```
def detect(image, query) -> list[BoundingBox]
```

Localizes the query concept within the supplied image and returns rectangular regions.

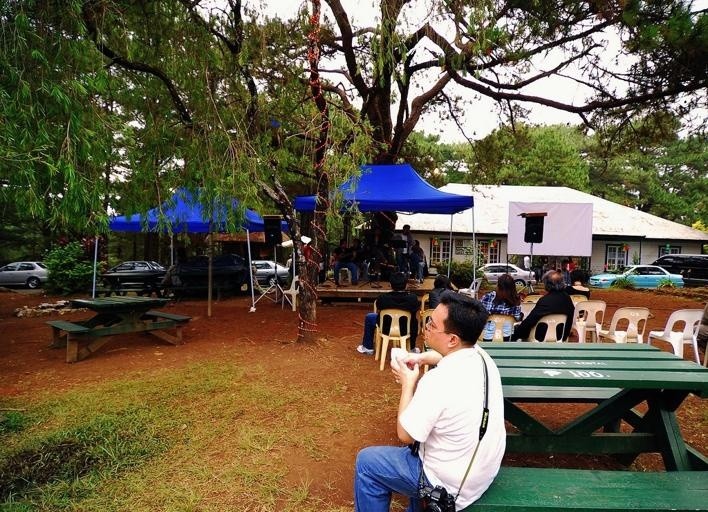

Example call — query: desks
[97,271,166,297]
[59,296,177,361]
[423,341,708,471]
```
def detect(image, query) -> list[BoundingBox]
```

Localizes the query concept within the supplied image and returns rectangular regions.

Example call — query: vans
[649,253,708,286]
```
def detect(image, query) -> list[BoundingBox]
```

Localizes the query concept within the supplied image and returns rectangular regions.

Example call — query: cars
[476,263,537,286]
[101,261,167,283]
[0,261,51,288]
[250,260,291,287]
[589,266,685,293]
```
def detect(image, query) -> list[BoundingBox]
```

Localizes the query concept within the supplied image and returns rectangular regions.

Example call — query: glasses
[428,317,454,334]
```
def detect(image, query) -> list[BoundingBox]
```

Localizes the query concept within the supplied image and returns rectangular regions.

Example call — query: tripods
[517,243,537,293]
[252,244,293,307]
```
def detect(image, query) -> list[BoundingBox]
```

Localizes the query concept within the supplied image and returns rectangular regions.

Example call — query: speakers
[524,216,544,243]
[263,216,283,244]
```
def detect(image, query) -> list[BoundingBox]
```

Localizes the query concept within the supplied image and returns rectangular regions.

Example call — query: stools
[339,267,352,285]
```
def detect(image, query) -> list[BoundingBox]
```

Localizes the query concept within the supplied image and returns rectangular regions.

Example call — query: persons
[285,225,591,356]
[693,302,708,355]
[355,288,507,512]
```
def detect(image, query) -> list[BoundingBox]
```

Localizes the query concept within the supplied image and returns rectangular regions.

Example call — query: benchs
[121,282,170,288]
[459,467,708,512]
[501,384,622,404]
[87,288,152,297]
[44,320,90,363]
[146,311,192,345]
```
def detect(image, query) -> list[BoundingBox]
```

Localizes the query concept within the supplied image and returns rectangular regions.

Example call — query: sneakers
[357,345,374,355]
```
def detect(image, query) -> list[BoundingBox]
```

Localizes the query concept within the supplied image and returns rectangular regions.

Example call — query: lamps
[489,240,494,247]
[434,237,438,245]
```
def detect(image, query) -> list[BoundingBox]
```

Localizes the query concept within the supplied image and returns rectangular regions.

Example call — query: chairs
[571,300,607,342]
[527,314,567,342]
[420,309,434,332]
[414,279,588,310]
[281,275,300,311]
[479,314,516,343]
[519,302,535,320]
[600,306,650,344]
[373,309,411,372]
[651,309,704,365]
